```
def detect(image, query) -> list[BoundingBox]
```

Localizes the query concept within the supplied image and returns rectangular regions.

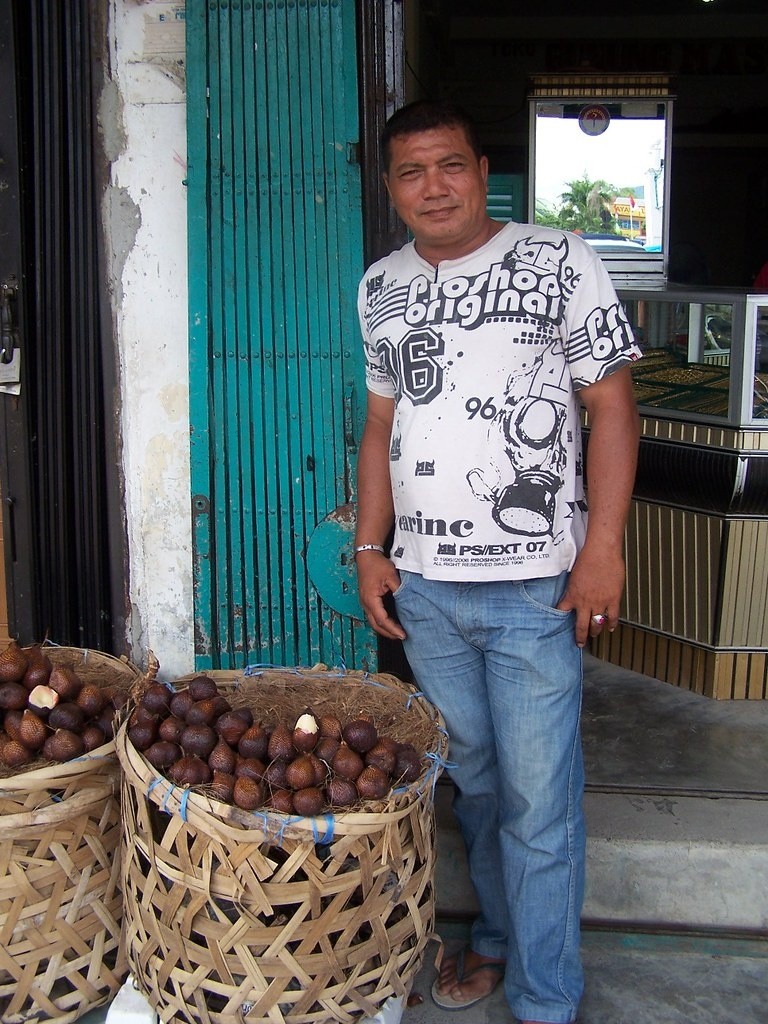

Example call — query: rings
[592,615,604,625]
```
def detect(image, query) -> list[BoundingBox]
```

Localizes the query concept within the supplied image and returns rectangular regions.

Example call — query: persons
[355,98,642,1024]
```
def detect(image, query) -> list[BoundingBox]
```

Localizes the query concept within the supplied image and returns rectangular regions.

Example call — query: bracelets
[354,544,385,555]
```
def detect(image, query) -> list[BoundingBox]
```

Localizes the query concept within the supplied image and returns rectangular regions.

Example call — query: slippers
[430,943,507,1010]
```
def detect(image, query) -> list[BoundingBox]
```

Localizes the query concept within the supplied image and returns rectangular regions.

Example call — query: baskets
[0,646,143,1024]
[120,667,448,1024]
